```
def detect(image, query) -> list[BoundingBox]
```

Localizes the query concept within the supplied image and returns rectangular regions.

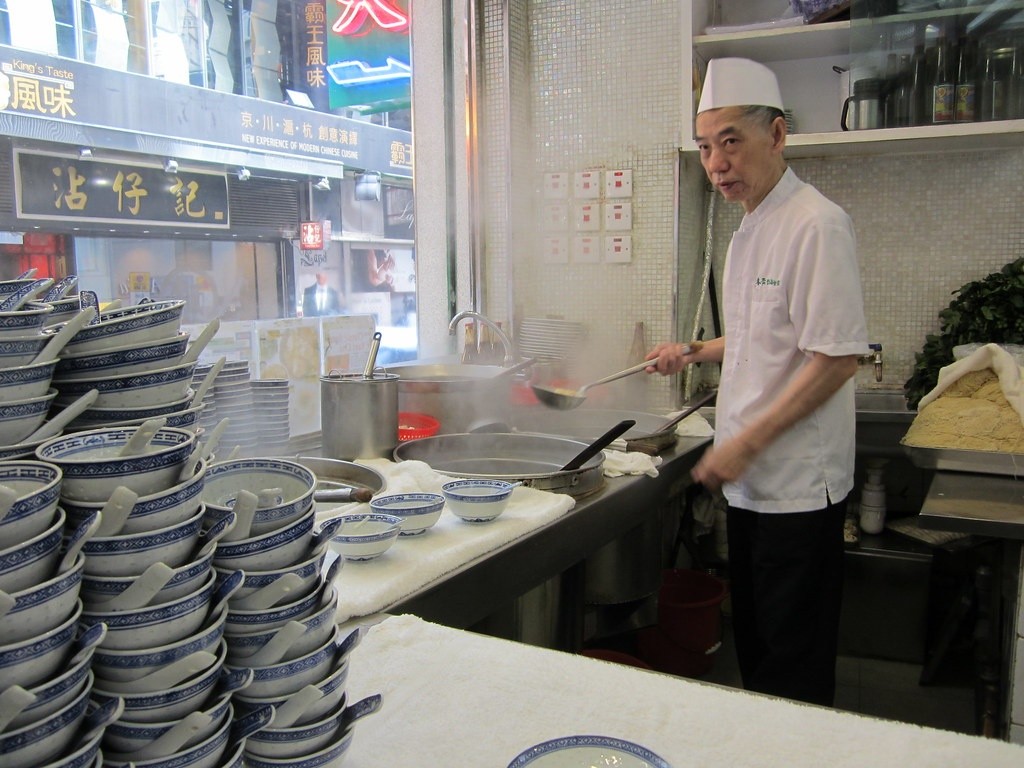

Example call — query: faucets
[446,309,517,368]
[870,343,886,382]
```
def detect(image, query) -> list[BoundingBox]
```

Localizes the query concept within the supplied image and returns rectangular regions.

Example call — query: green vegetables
[902,256,1024,412]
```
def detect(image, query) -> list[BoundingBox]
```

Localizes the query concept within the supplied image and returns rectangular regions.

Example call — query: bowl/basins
[0,277,669,768]
[839,65,878,130]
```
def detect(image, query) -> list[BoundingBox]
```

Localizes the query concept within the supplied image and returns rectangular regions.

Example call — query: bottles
[887,36,1024,126]
[493,321,503,366]
[625,321,648,408]
[460,321,479,366]
[478,322,494,365]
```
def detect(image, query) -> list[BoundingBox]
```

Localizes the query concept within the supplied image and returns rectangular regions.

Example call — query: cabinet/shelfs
[681,0,1024,163]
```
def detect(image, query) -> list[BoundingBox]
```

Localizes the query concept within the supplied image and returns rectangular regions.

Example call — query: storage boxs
[836,511,1001,668]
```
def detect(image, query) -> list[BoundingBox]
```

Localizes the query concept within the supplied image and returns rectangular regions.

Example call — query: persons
[642,57,871,710]
[352,249,399,292]
[302,270,340,316]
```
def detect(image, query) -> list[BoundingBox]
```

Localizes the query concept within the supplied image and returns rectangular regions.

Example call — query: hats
[697,57,785,115]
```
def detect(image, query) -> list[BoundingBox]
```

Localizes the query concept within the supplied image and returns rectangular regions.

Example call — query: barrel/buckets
[580,649,654,671]
[637,568,723,676]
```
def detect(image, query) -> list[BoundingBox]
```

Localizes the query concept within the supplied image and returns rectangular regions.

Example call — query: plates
[519,316,585,389]
[783,107,794,133]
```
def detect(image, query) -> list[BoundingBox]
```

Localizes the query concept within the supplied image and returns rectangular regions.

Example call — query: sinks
[684,389,926,514]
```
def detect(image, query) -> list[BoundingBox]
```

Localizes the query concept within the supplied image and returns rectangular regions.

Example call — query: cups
[840,77,886,131]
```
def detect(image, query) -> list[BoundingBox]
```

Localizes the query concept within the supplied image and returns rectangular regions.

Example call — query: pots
[374,363,513,423]
[319,365,400,462]
[392,431,606,501]
[511,408,678,455]
